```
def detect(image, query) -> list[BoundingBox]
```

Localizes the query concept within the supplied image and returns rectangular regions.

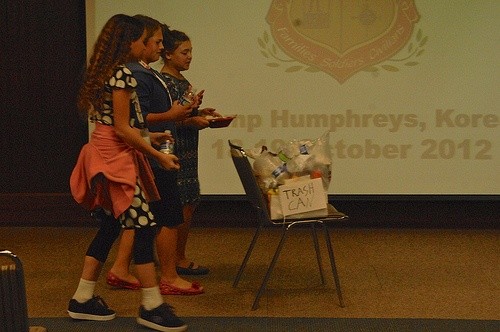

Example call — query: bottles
[256,153,289,185]
[159,130,173,169]
[178,88,196,106]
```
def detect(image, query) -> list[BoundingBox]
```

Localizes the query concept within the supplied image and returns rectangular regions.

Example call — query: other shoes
[160,278,204,295]
[104,270,140,290]
[176,261,210,274]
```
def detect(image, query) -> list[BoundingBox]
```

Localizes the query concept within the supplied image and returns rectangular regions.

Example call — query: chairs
[229,139,350,312]
[0,251,47,332]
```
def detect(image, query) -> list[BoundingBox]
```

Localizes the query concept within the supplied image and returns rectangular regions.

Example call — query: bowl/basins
[206,117,236,128]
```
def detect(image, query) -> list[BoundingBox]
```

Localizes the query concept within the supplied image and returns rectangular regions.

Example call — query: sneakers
[136,303,187,331]
[67,296,116,321]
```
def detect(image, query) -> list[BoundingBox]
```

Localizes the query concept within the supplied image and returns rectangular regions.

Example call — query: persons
[105,15,205,296]
[66,14,188,332]
[133,22,222,276]
